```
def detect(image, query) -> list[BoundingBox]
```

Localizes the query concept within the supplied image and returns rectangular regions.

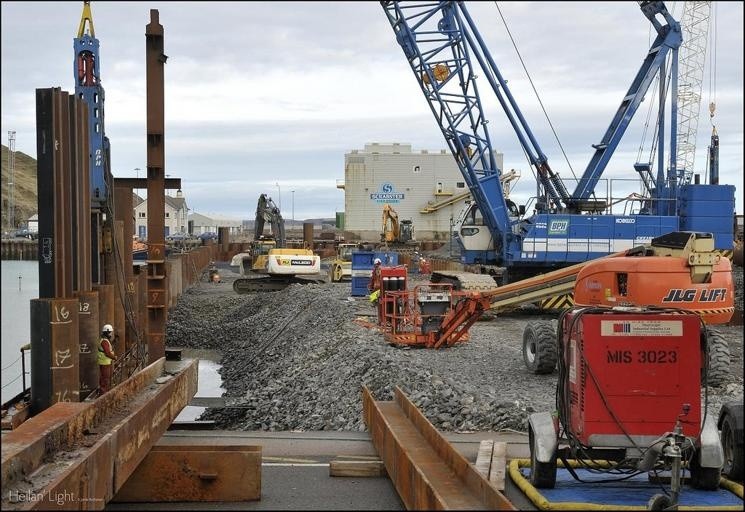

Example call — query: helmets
[374,258,382,265]
[102,324,114,332]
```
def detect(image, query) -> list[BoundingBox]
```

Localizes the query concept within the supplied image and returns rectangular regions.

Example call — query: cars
[166,231,190,242]
[195,232,217,240]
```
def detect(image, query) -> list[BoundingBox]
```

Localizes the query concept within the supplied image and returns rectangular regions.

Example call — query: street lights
[275,184,282,211]
[134,168,140,205]
[290,189,294,234]
[166,174,170,195]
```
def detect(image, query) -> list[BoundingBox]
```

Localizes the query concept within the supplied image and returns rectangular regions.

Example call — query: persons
[370,257,382,294]
[99,323,118,397]
[410,251,419,275]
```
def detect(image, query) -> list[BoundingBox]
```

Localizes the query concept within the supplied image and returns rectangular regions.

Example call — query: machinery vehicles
[329,243,367,283]
[376,0,745,511]
[232,193,327,295]
